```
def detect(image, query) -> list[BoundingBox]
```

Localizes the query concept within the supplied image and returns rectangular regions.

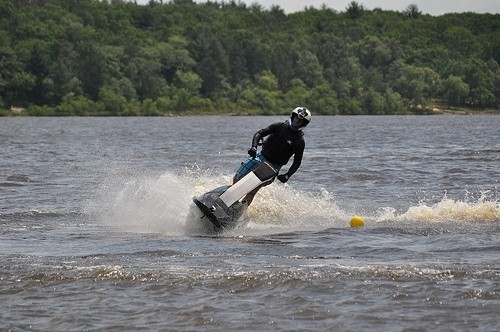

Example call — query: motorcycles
[193,149,286,231]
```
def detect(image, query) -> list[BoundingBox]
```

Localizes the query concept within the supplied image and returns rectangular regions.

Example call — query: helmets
[289,106,311,130]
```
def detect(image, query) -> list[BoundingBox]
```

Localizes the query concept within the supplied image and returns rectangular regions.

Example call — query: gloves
[248,146,257,160]
[277,174,288,183]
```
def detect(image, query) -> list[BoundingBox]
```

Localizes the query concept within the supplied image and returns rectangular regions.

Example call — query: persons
[233,107,311,209]
